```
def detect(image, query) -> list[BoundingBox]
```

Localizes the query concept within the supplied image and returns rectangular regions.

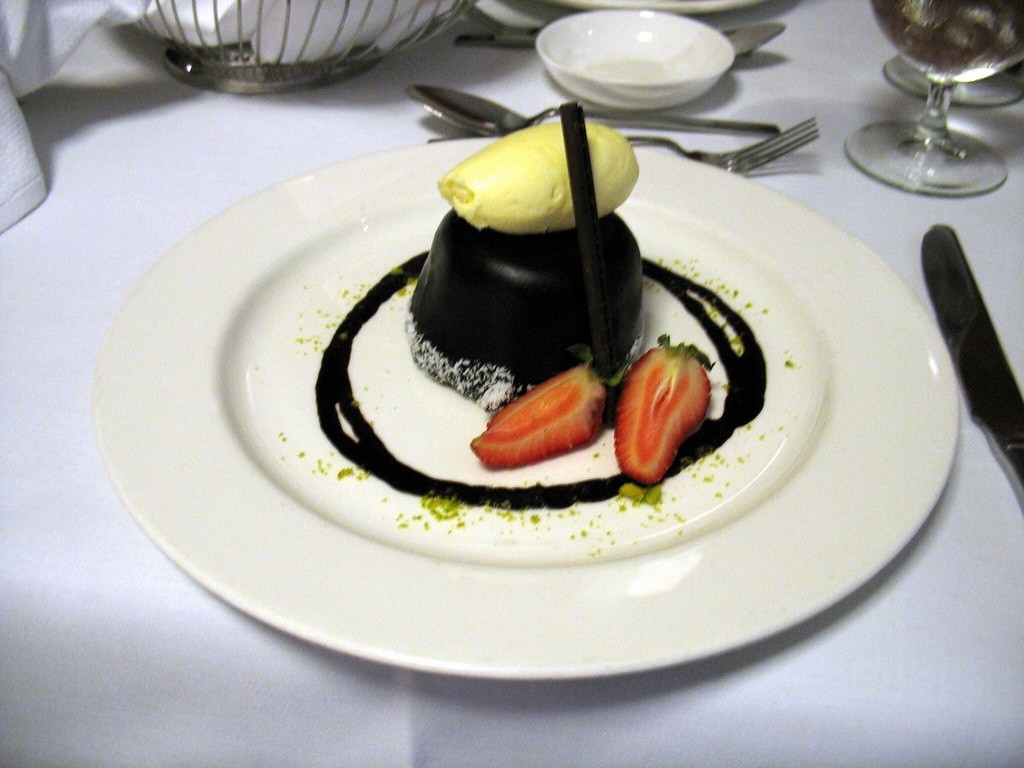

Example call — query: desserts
[405,101,645,414]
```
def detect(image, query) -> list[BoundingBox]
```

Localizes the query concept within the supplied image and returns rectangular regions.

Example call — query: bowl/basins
[535,9,735,107]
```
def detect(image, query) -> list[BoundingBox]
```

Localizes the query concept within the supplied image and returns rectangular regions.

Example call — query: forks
[627,116,819,171]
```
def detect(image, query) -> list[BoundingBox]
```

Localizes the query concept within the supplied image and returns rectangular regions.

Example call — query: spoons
[455,22,785,58]
[408,85,779,142]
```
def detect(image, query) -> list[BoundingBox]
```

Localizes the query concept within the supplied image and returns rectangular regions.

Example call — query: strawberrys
[469,344,635,469]
[615,333,716,484]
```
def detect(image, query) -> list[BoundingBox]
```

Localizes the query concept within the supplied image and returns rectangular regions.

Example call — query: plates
[92,140,959,683]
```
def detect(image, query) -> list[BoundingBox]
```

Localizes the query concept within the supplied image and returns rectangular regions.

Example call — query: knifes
[920,223,1024,500]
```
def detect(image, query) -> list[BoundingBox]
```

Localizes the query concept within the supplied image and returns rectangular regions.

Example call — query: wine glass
[844,0,1024,196]
[881,54,1024,109]
